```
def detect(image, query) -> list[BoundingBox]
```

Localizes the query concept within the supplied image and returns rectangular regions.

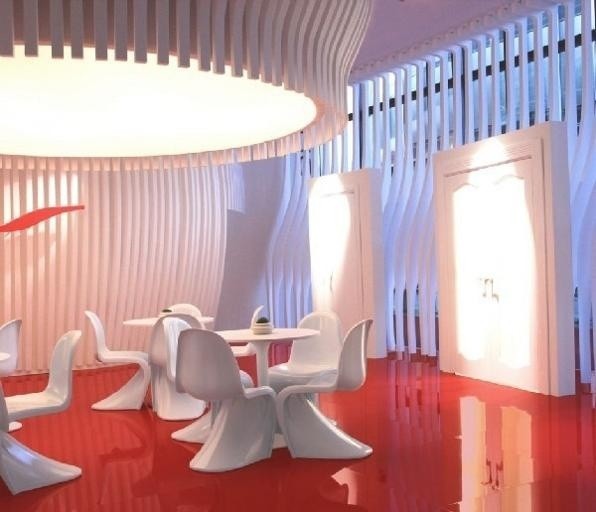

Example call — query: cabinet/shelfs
[317,361,391,508]
[450,391,550,509]
[433,121,575,397]
[308,167,387,359]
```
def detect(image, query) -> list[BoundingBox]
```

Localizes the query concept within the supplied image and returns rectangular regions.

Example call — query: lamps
[2,0,374,172]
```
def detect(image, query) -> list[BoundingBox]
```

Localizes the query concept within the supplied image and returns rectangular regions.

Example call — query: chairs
[148,313,208,421]
[84,309,151,410]
[1,318,23,431]
[229,305,268,358]
[1,329,83,495]
[274,318,374,461]
[170,328,277,473]
[266,309,346,426]
[160,303,201,317]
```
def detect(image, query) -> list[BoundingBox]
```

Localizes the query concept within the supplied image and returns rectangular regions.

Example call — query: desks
[213,326,321,451]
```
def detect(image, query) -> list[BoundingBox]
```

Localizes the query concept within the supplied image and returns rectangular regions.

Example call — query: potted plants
[252,317,272,334]
